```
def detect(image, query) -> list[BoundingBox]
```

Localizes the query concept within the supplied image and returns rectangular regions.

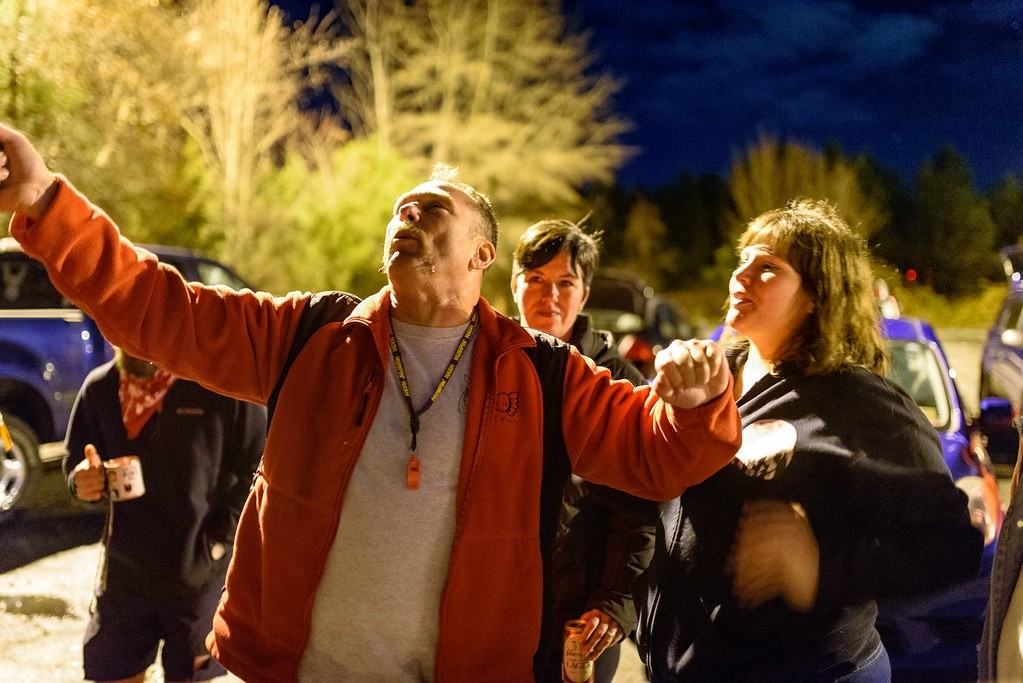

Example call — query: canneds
[561,620,596,683]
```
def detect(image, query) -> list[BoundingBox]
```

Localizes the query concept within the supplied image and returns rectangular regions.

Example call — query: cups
[98,456,145,502]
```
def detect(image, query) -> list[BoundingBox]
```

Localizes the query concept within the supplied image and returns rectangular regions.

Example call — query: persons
[632,194,985,683]
[0,122,744,683]
[503,214,657,683]
[974,393,1023,683]
[63,337,267,683]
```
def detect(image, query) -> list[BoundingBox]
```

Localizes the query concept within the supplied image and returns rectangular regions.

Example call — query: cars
[579,274,701,379]
[876,317,1020,682]
[979,283,1023,423]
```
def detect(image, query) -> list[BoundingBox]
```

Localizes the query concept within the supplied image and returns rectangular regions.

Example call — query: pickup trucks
[0,236,260,525]
